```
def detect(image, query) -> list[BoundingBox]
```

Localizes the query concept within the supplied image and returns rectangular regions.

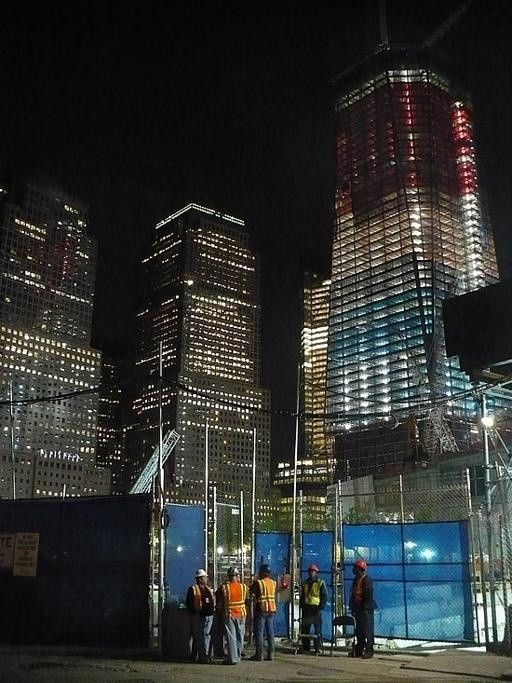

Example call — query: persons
[186,567,216,664]
[215,567,251,665]
[347,561,378,659]
[296,564,328,654]
[249,564,278,661]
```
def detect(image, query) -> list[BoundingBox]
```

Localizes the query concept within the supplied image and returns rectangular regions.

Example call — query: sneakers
[249,653,274,661]
[293,646,322,654]
[197,657,236,665]
[348,649,373,660]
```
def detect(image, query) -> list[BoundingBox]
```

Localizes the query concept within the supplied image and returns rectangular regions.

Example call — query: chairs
[293,615,356,657]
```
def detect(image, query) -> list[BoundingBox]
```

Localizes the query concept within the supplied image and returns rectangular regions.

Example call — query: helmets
[355,560,367,570]
[260,565,271,572]
[195,568,208,577]
[308,564,318,571]
[227,567,240,575]
